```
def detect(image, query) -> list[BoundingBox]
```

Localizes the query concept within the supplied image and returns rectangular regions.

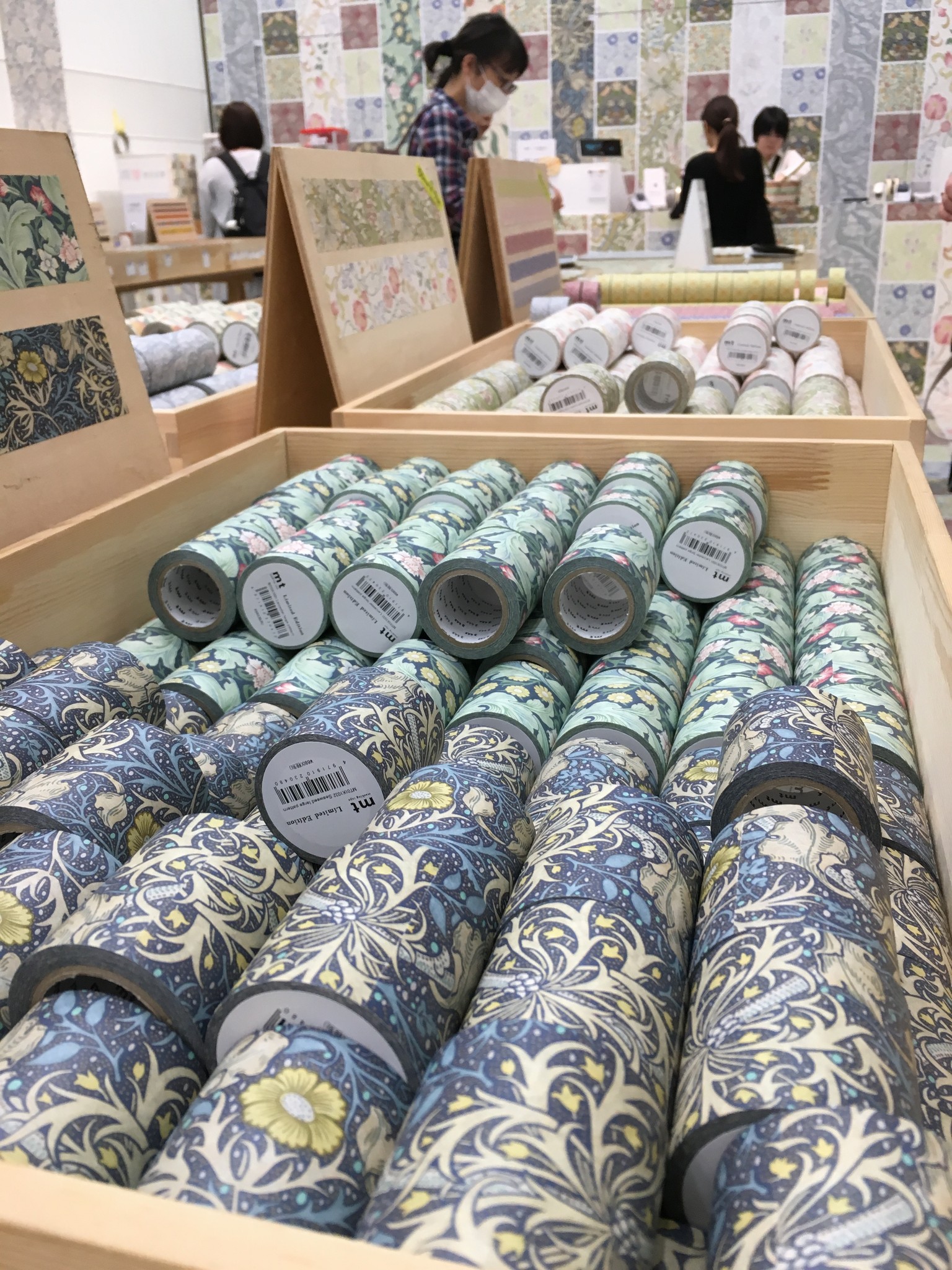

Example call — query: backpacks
[214,150,270,237]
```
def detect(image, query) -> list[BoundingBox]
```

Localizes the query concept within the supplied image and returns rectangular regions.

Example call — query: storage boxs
[0,128,952,1270]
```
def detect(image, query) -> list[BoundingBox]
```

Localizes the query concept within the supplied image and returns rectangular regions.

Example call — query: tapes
[124,300,266,408]
[0,451,949,1270]
[416,265,860,417]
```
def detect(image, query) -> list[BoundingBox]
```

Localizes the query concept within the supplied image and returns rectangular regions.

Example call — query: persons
[667,95,791,246]
[194,102,273,305]
[409,11,530,270]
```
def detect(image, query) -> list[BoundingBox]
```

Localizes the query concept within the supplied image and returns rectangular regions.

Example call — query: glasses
[483,57,518,96]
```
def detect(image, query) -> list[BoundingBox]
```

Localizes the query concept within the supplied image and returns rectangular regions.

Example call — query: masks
[463,78,509,116]
